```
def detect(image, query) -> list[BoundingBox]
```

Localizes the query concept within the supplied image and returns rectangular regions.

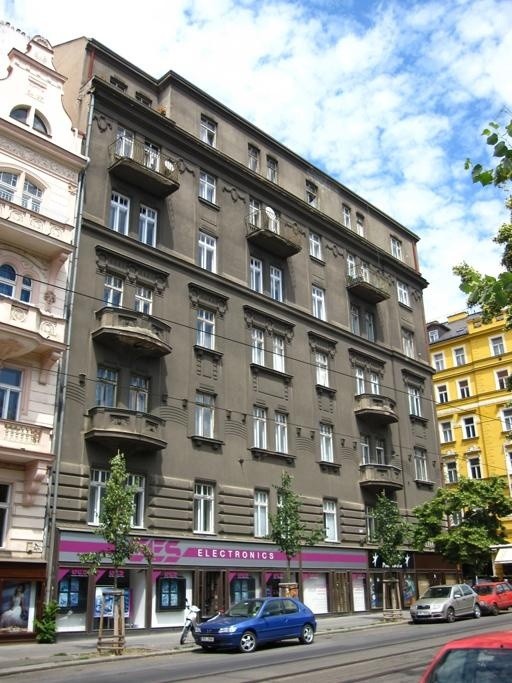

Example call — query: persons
[0,584,27,628]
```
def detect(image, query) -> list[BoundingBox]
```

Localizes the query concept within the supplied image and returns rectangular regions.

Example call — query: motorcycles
[177,596,223,645]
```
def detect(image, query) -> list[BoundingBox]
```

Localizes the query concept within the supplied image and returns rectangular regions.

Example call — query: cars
[419,631,512,682]
[410,582,481,623]
[470,580,512,616]
[195,598,317,652]
